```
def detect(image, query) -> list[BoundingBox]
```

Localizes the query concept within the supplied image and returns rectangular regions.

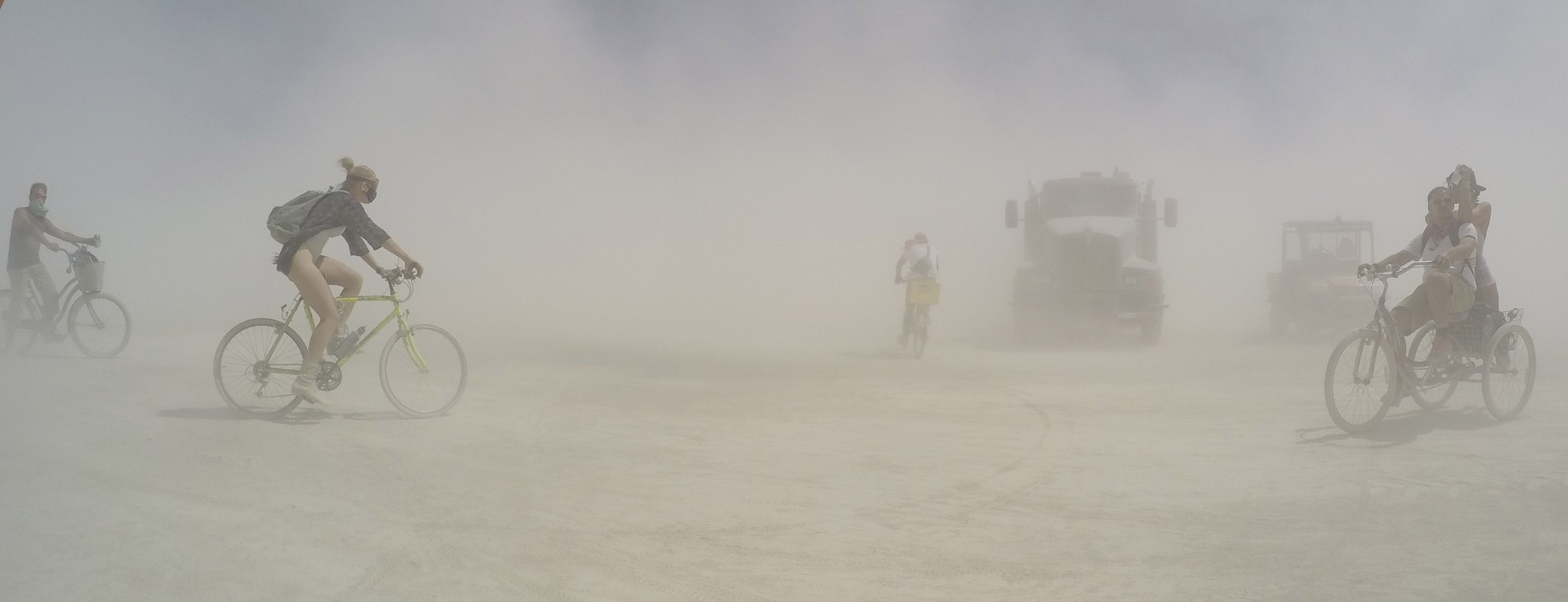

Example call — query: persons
[1357,163,1512,403]
[276,156,423,407]
[0,182,101,350]
[894,232,939,351]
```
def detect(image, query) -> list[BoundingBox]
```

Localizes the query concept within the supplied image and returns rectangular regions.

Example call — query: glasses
[1429,196,1453,208]
[346,175,379,190]
[1449,184,1454,193]
[33,193,47,200]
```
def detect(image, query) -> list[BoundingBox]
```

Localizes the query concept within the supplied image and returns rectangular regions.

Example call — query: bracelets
[1372,262,1380,272]
[376,266,384,274]
[1461,181,1471,189]
[47,241,51,249]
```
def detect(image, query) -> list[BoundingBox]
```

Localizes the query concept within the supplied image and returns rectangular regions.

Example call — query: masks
[364,183,377,203]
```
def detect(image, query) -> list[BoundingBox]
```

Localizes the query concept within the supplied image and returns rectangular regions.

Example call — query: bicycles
[0,243,132,361]
[212,266,469,419]
[897,277,941,357]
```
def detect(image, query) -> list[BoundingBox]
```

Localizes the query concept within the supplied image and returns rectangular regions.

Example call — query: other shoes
[1494,353,1512,370]
[1426,338,1453,365]
[1380,370,1418,403]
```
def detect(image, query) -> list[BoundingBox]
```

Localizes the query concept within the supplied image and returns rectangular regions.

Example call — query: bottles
[334,325,365,360]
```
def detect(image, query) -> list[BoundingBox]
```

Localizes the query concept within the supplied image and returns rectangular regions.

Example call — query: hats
[1447,168,1487,191]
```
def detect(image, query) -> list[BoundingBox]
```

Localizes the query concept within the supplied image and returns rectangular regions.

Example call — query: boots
[291,358,330,407]
[327,319,357,356]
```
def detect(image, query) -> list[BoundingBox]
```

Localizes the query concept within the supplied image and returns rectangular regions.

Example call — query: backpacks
[266,186,351,245]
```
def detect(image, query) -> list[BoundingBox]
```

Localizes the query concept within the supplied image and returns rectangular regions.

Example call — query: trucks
[1002,172,1179,344]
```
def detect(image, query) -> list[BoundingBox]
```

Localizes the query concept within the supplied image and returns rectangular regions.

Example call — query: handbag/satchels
[912,257,933,274]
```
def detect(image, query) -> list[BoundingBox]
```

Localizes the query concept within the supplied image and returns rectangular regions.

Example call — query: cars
[1266,221,1383,332]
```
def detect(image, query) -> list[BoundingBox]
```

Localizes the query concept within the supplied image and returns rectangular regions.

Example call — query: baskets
[1430,309,1524,357]
[73,262,105,291]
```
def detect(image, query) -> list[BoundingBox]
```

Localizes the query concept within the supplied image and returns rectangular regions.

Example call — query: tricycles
[1323,261,1538,435]
